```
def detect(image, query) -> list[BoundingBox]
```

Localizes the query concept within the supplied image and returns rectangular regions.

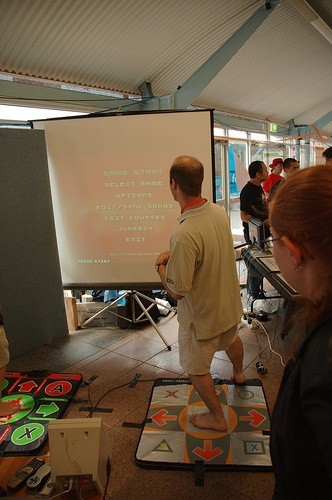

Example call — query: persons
[263,158,284,200]
[322,147,332,165]
[271,158,300,198]
[155,156,245,432]
[0,312,21,417]
[265,165,332,500]
[238,161,272,308]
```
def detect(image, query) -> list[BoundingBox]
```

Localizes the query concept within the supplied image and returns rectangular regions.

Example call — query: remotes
[26,463,51,488]
[8,457,45,491]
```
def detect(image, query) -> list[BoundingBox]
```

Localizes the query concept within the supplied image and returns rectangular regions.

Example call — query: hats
[269,158,283,168]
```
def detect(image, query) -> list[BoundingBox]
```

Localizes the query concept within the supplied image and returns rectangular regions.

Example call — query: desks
[241,248,298,313]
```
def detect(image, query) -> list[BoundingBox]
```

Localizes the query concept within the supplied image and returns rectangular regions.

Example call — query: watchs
[155,263,166,273]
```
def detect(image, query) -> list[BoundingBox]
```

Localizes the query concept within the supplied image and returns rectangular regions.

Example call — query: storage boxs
[76,302,118,327]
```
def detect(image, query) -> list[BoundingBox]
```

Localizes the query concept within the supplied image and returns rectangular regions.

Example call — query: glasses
[263,233,314,261]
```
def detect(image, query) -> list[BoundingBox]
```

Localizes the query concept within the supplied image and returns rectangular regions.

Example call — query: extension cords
[241,316,259,329]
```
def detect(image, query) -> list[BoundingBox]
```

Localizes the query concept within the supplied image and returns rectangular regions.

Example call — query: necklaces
[181,198,204,214]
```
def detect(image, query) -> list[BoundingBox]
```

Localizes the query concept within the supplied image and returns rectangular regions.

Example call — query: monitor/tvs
[48,418,111,500]
[249,217,265,251]
